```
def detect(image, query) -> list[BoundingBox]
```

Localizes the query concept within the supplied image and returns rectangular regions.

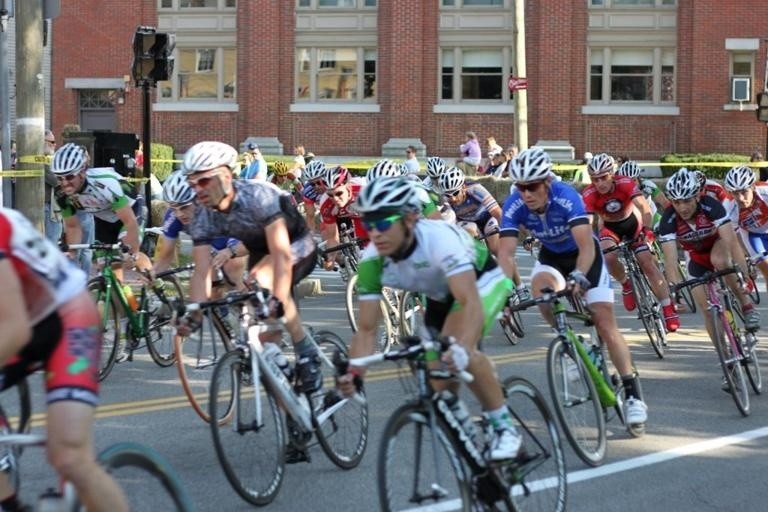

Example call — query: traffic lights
[131,27,157,84]
[755,93,768,123]
[152,33,177,82]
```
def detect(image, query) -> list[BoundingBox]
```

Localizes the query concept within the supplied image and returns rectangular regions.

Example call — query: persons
[1,131,768,512]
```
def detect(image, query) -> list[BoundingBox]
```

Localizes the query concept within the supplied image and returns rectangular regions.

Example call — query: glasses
[45,139,55,144]
[326,192,344,198]
[167,201,193,212]
[361,214,403,232]
[406,151,413,153]
[55,172,80,183]
[443,187,462,198]
[188,171,224,188]
[278,174,287,177]
[309,179,322,187]
[590,173,611,183]
[513,181,545,192]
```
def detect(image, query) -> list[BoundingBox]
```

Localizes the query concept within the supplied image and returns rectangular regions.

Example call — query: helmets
[322,165,349,192]
[302,160,327,181]
[618,160,641,180]
[181,140,239,177]
[693,171,707,187]
[723,165,757,193]
[352,176,423,218]
[426,157,446,179]
[507,147,552,184]
[161,169,197,204]
[50,142,89,174]
[587,153,614,178]
[365,159,408,183]
[439,165,465,193]
[664,168,701,201]
[273,159,289,176]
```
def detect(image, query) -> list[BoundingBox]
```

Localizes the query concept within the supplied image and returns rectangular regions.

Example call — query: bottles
[439,387,478,441]
[120,283,139,312]
[213,306,242,337]
[723,309,742,340]
[263,341,296,384]
[578,334,606,375]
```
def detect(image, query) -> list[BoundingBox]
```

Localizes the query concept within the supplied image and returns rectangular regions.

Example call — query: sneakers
[622,394,649,424]
[515,284,531,304]
[483,426,527,462]
[114,337,133,364]
[563,349,583,383]
[621,277,637,311]
[742,305,762,332]
[147,286,165,316]
[293,343,324,395]
[661,298,681,332]
[283,436,307,464]
[720,375,738,394]
